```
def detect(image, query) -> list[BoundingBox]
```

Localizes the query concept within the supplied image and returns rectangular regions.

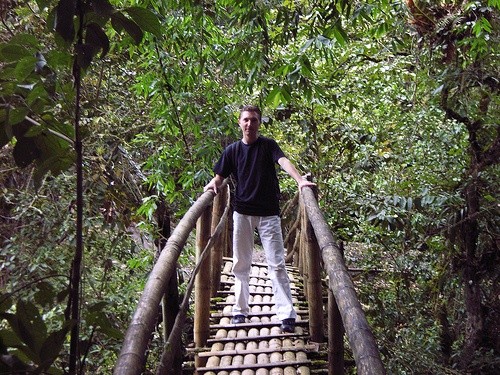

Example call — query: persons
[203,106,318,332]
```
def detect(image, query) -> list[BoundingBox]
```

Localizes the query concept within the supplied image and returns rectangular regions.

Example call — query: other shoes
[232,316,246,325]
[280,317,296,332]
